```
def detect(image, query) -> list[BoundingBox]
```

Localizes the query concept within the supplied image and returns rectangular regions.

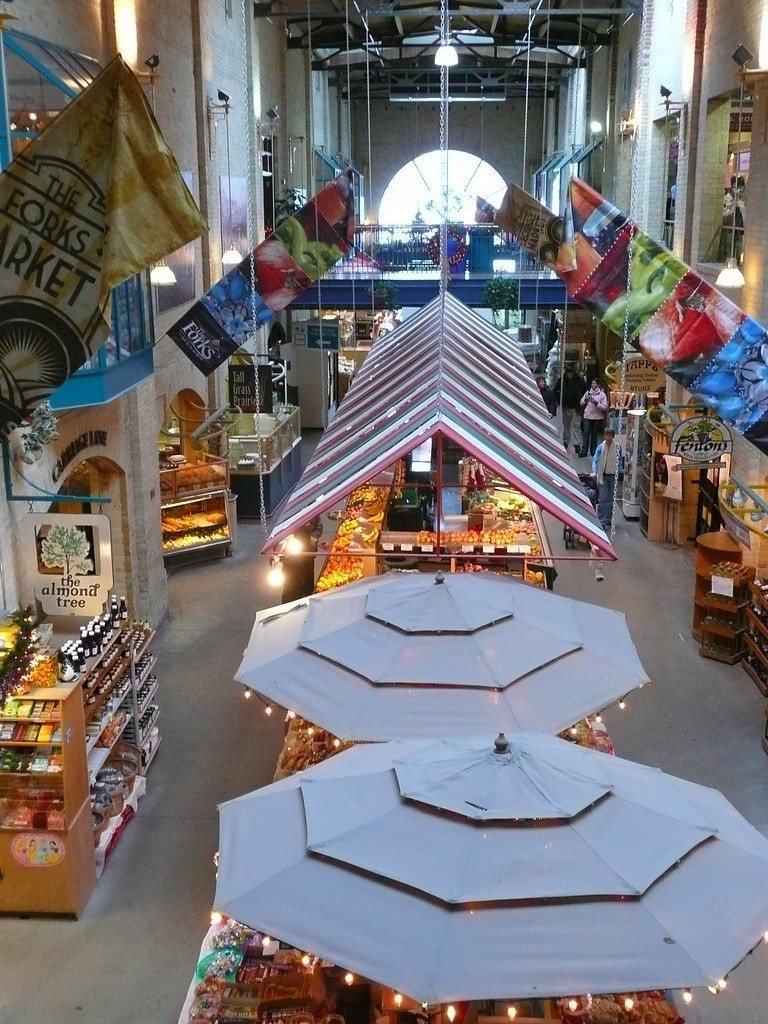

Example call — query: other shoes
[601,522,606,529]
[579,453,587,457]
[575,445,581,453]
[564,444,568,448]
[606,520,611,526]
[591,452,595,456]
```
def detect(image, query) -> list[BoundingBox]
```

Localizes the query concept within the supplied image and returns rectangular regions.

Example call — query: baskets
[91,742,142,849]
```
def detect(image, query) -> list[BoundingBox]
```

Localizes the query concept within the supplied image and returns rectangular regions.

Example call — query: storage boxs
[0,799,65,831]
[28,656,59,688]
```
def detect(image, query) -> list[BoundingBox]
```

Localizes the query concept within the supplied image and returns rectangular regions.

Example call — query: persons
[536,376,557,419]
[553,365,586,454]
[590,427,624,530]
[654,456,668,484]
[723,176,745,265]
[578,377,608,458]
[665,185,677,227]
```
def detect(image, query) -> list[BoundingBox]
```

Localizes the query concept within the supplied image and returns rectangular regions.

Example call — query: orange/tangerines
[335,531,351,552]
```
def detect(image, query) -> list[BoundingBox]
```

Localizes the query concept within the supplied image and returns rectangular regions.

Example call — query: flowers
[21,399,61,452]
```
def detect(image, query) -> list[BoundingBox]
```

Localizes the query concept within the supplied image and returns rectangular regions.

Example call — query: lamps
[145,54,159,72]
[716,78,746,290]
[733,43,754,70]
[267,108,280,123]
[146,76,178,286]
[222,109,245,264]
[216,88,230,105]
[435,0,459,66]
[660,85,673,102]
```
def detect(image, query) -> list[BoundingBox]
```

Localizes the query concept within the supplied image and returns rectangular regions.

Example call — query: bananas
[363,487,386,543]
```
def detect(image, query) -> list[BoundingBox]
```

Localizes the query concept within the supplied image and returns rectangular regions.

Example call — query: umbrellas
[213,733,767,1005]
[234,567,654,741]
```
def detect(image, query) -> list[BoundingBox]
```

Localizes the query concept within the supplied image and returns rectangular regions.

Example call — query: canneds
[86,625,157,740]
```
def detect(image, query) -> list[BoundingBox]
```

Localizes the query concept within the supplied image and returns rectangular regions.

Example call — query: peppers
[289,217,344,280]
[602,234,687,342]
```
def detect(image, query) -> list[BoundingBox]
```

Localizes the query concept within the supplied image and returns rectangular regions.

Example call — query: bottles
[57,595,158,738]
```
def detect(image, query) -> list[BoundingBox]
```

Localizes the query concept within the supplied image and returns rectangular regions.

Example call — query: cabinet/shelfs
[639,423,670,544]
[0,681,99,923]
[690,531,768,697]
[61,615,161,871]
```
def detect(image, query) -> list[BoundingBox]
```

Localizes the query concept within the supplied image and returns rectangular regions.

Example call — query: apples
[317,556,363,592]
[418,530,479,543]
